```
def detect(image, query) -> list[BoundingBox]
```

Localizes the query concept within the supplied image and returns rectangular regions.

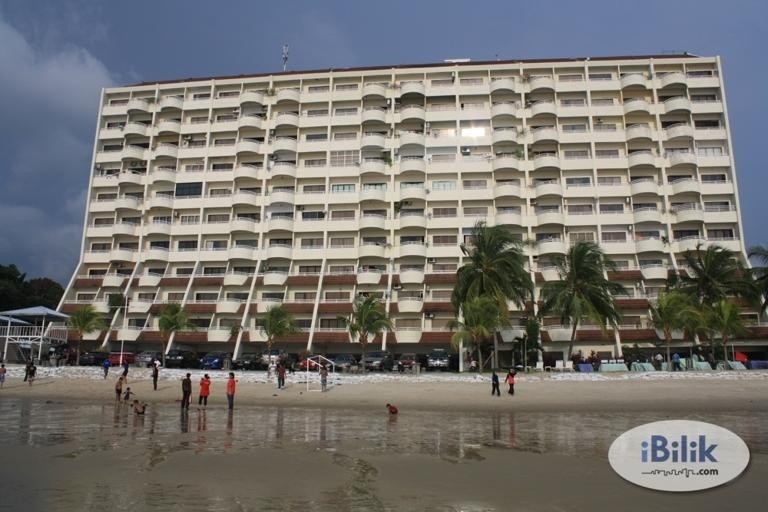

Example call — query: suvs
[398,352,417,371]
[425,348,452,371]
[48,344,359,373]
[359,351,394,371]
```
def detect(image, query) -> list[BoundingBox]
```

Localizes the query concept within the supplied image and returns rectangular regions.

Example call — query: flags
[735,351,746,362]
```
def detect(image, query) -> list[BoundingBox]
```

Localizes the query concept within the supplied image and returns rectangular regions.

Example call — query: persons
[23,358,32,381]
[225,410,235,450]
[289,359,296,374]
[226,371,235,409]
[319,365,329,391]
[507,410,519,449]
[150,363,159,391]
[112,409,157,442]
[491,371,500,395]
[133,399,147,414]
[386,403,398,414]
[114,376,123,404]
[102,358,112,379]
[179,408,191,446]
[504,368,517,394]
[268,360,276,379]
[470,359,477,370]
[120,358,130,384]
[121,387,137,405]
[276,364,286,389]
[196,409,206,453]
[130,401,143,411]
[0,364,7,386]
[153,357,161,370]
[672,351,681,370]
[26,362,38,383]
[692,352,698,361]
[197,373,211,410]
[489,411,503,443]
[654,352,663,370]
[465,351,472,364]
[705,351,715,363]
[181,372,193,407]
